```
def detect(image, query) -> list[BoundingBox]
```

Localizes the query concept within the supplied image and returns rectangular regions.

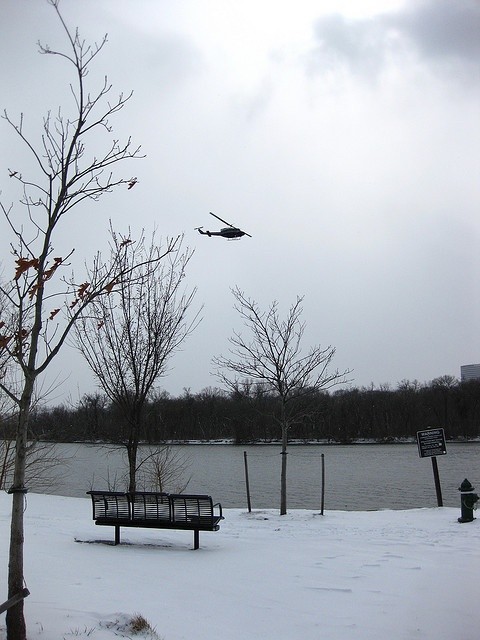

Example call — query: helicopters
[194,213,252,241]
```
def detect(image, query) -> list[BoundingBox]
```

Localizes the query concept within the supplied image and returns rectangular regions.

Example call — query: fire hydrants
[457,476,479,523]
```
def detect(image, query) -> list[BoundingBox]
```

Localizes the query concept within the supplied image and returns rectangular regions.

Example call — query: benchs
[86,491,224,550]
